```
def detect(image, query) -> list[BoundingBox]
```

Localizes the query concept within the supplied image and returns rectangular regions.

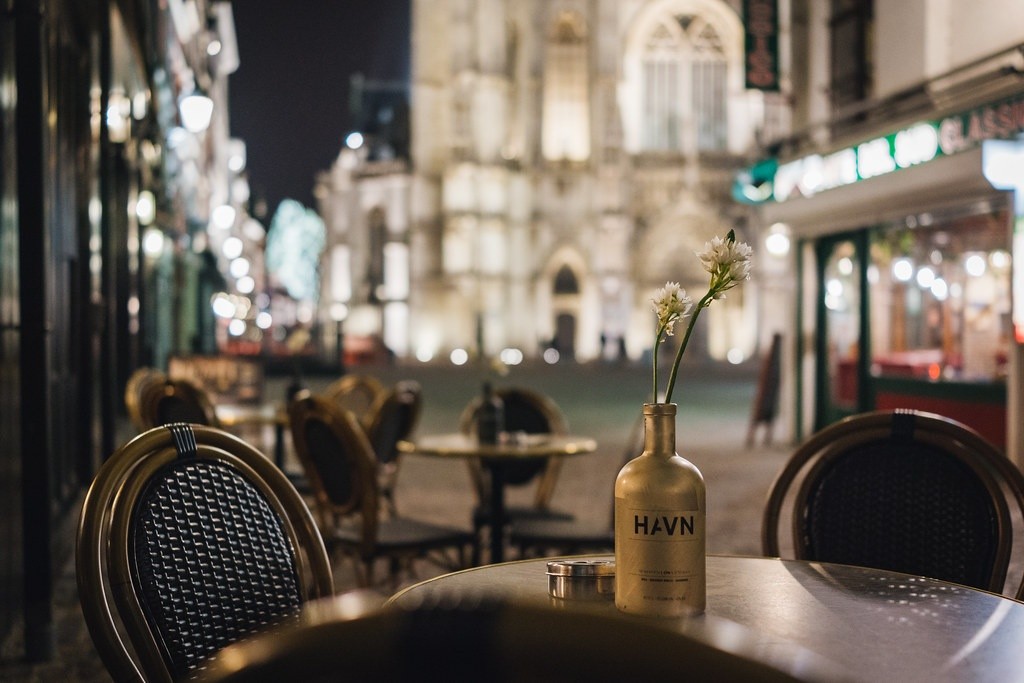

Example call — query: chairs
[761,405,1024,601]
[70,425,339,683]
[123,363,637,580]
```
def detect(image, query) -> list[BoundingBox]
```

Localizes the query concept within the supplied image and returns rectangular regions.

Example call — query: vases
[612,401,711,618]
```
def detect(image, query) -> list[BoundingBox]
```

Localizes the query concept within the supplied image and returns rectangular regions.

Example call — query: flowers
[645,233,756,405]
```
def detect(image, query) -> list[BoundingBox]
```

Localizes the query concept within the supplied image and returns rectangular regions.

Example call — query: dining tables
[372,545,1024,683]
